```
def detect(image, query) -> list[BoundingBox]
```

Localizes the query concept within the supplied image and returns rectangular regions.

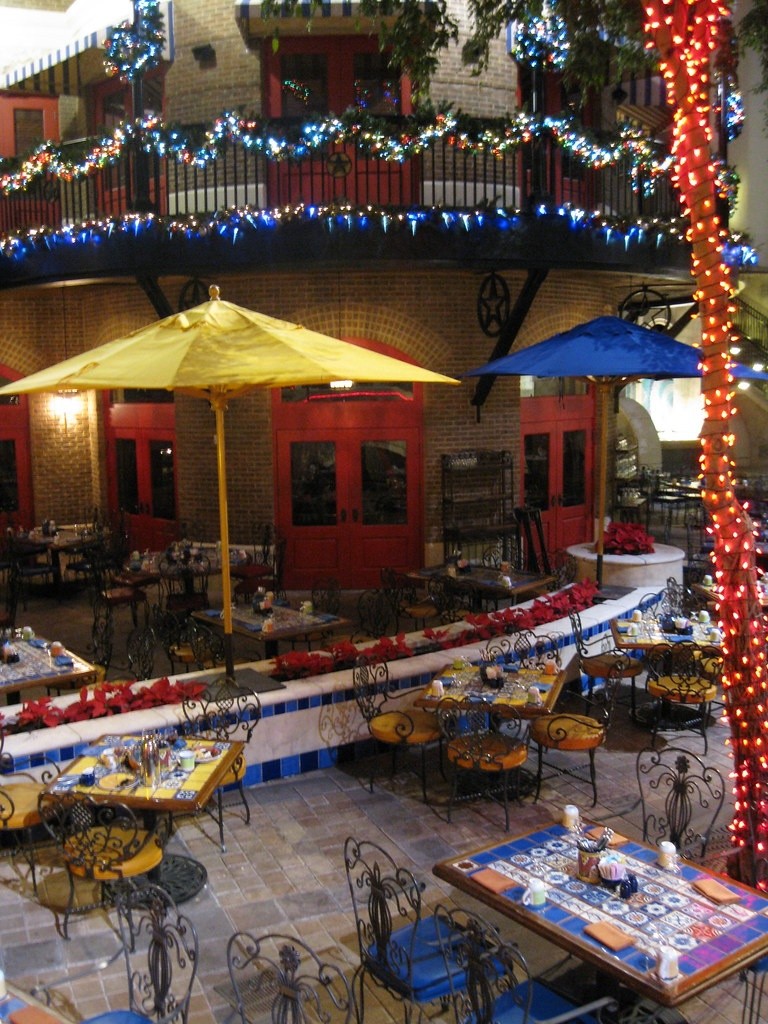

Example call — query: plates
[176,748,221,763]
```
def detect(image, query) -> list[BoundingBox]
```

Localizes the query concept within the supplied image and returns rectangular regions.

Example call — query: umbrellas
[455,304,767,590]
[0,285,462,686]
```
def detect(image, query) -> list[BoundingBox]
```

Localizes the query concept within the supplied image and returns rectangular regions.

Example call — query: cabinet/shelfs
[439,448,518,564]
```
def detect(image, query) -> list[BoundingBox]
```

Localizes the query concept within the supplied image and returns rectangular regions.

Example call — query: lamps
[191,45,214,61]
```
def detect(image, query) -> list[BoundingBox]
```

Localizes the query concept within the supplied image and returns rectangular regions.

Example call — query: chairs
[0,522,767,1024]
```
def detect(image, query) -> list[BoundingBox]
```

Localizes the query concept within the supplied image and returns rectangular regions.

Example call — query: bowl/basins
[489,679,503,688]
[675,625,693,635]
[601,876,623,889]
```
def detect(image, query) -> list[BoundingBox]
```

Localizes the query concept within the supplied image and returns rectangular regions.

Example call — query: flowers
[0,512,654,737]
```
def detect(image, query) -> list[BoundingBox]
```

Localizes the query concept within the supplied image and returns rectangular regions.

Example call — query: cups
[703,575,712,586]
[620,875,638,898]
[479,660,496,684]
[627,622,637,636]
[576,845,605,883]
[563,805,578,826]
[78,739,196,787]
[655,946,682,979]
[453,655,464,669]
[19,626,63,656]
[633,610,641,621]
[432,680,444,696]
[544,659,556,675]
[662,613,675,633]
[528,686,539,703]
[710,628,723,641]
[521,878,545,906]
[27,517,57,538]
[699,611,709,622]
[658,840,675,867]
[130,547,150,570]
[673,478,677,484]
[253,584,315,632]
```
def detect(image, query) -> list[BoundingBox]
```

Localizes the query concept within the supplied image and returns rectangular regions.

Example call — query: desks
[19,533,98,597]
[154,567,221,615]
[613,498,649,534]
[690,577,768,617]
[412,658,567,813]
[434,816,768,1024]
[191,603,345,667]
[39,737,248,910]
[611,619,725,729]
[408,564,557,626]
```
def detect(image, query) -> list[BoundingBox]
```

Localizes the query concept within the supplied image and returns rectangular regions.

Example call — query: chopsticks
[574,827,614,853]
